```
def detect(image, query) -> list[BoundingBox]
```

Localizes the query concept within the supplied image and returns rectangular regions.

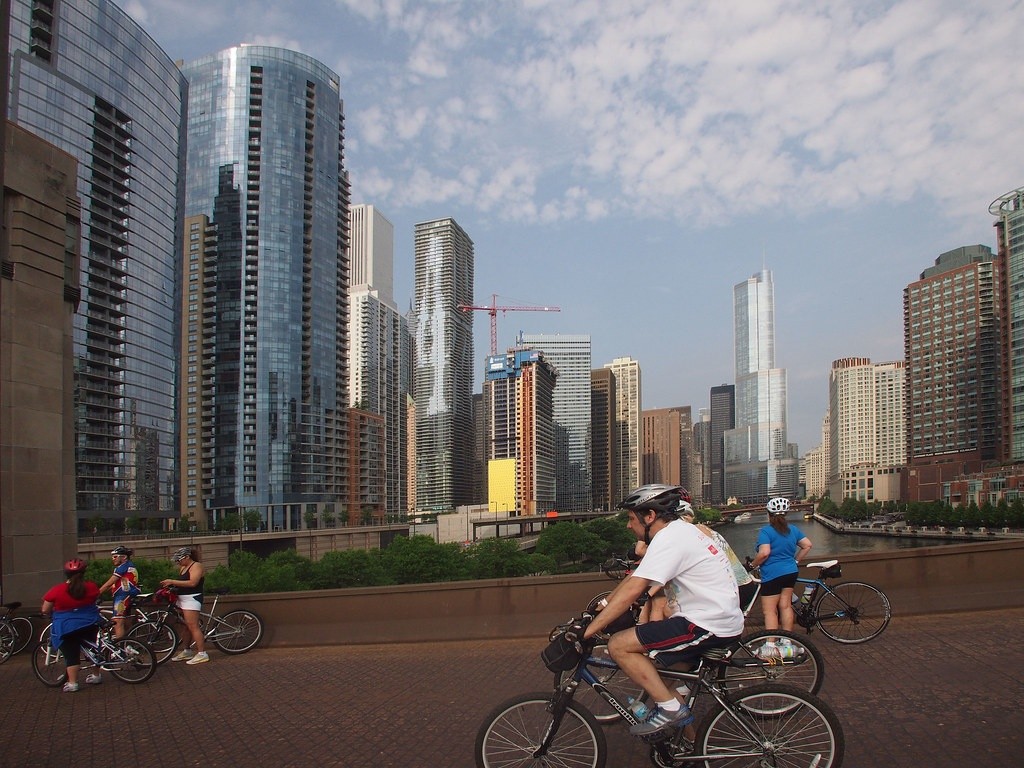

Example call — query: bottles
[800,584,814,604]
[627,696,652,722]
[758,646,805,658]
[790,592,801,609]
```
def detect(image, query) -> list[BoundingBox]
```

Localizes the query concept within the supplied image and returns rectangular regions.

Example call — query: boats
[734,511,751,523]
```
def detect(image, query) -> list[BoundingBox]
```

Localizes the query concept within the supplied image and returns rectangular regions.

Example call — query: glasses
[177,556,186,563]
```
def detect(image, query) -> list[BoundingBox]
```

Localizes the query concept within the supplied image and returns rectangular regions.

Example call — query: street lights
[490,501,497,521]
[502,503,508,520]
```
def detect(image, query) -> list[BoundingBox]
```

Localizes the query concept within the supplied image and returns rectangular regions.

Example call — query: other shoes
[185,651,209,664]
[170,650,195,662]
[85,673,103,684]
[62,680,79,692]
[676,685,692,702]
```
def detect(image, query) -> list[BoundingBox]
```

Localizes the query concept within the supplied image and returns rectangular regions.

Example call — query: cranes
[456,294,561,356]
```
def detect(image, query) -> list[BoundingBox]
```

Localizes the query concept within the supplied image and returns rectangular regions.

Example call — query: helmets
[172,547,191,562]
[676,486,690,503]
[677,500,695,518]
[110,545,134,556]
[64,557,86,573]
[766,497,791,515]
[615,483,683,509]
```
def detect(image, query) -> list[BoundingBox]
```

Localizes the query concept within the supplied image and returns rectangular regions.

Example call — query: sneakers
[628,702,694,738]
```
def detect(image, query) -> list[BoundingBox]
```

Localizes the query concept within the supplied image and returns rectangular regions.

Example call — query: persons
[96,546,138,660]
[746,498,812,655]
[40,558,102,692]
[159,548,210,664]
[565,484,755,768]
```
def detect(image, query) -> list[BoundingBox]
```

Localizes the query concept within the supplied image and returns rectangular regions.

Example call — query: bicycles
[471,557,893,768]
[0,586,265,689]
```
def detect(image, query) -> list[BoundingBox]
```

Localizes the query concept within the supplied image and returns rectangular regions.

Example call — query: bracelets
[795,559,798,563]
[600,598,608,608]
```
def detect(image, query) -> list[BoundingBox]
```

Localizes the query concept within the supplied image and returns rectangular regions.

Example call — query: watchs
[748,563,755,569]
